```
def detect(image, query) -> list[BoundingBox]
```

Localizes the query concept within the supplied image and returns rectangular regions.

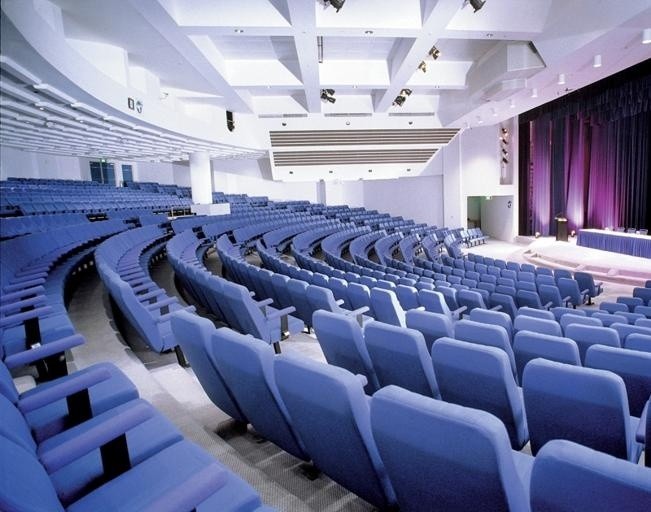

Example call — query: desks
[576,228,651,259]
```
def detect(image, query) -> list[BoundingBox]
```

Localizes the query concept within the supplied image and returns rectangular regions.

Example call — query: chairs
[0,177,275,511]
[490,256,651,512]
[604,225,651,236]
[275,201,489,512]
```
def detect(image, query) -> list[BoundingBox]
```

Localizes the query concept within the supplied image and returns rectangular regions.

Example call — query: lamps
[470,0,488,13]
[325,0,345,12]
